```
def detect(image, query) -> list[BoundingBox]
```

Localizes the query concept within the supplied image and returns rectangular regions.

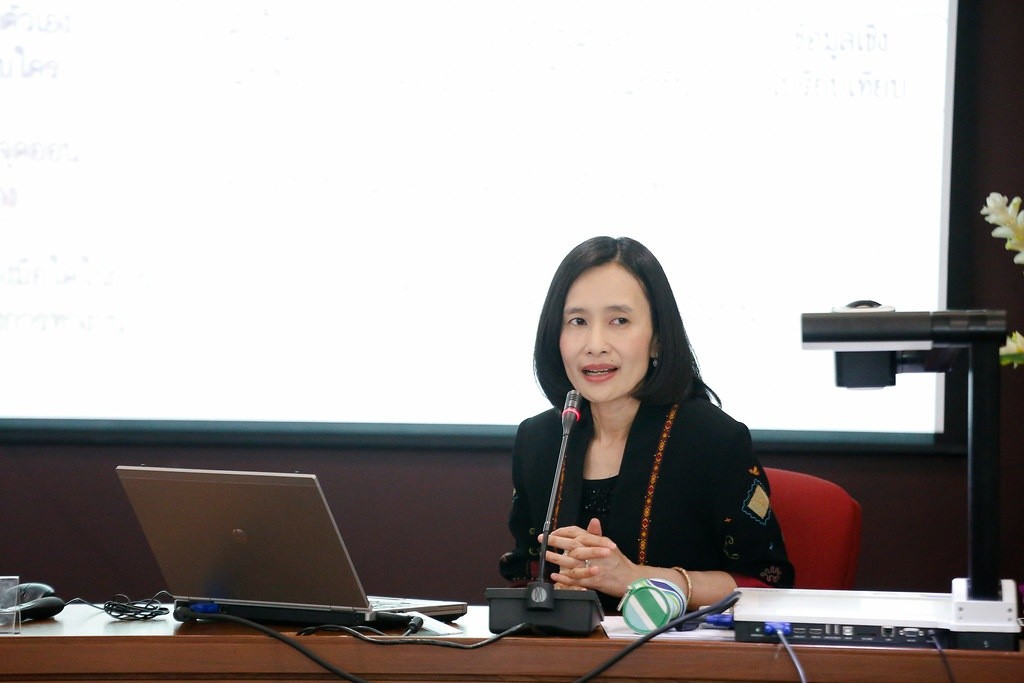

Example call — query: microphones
[485,390,607,637]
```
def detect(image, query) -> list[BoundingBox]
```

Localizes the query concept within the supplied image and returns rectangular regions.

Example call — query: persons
[500,235,796,617]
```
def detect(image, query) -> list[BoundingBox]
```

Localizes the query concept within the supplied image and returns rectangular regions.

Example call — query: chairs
[753,466,865,591]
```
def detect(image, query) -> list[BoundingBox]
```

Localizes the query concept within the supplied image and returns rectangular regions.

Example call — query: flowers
[978,188,1024,366]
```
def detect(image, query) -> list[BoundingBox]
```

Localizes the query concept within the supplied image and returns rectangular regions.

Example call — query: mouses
[1,582,56,610]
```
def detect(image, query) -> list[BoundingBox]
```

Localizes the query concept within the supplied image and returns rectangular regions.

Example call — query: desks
[0,599,1024,683]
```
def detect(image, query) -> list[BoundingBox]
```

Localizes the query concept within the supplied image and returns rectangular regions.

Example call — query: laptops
[115,464,468,627]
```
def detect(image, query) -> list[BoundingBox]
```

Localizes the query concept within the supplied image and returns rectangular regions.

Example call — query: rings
[585,558,590,569]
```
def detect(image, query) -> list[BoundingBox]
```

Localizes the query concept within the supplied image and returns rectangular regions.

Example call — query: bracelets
[671,566,693,605]
